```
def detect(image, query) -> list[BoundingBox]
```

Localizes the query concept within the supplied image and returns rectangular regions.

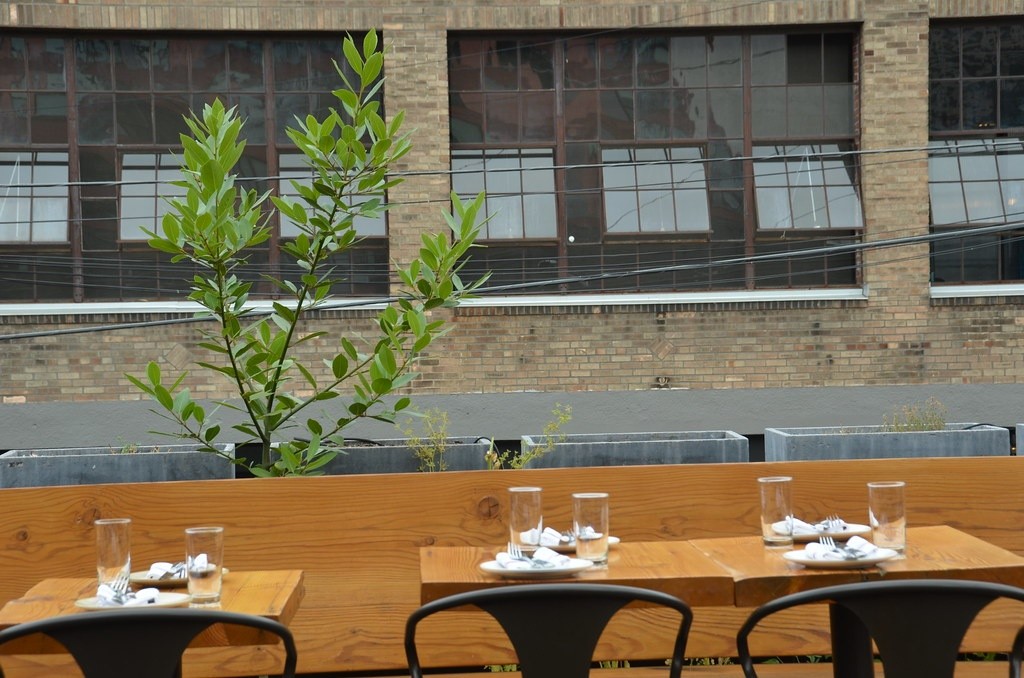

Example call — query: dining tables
[420,542,734,611]
[0,570,305,655]
[688,525,1024,608]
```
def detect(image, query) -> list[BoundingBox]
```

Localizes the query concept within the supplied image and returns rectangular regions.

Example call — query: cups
[571,490,608,562]
[94,518,130,592]
[184,525,225,598]
[189,599,221,611]
[573,562,608,579]
[866,480,906,554]
[508,486,544,551]
[757,476,792,548]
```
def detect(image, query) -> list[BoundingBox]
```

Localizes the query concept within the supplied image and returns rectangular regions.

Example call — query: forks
[819,535,855,560]
[560,529,574,544]
[506,540,537,567]
[826,512,843,536]
[159,562,184,580]
[110,573,128,603]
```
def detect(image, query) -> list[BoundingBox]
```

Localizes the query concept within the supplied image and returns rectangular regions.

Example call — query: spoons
[135,588,160,606]
[813,519,829,530]
[805,543,822,561]
[180,554,194,578]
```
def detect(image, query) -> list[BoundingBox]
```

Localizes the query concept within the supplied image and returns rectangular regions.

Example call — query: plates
[540,533,620,550]
[577,534,603,540]
[129,566,229,584]
[74,592,190,609]
[780,547,899,568]
[792,523,870,542]
[771,520,790,534]
[480,557,595,577]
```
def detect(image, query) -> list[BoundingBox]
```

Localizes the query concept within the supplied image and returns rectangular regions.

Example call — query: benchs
[0,456,1024,678]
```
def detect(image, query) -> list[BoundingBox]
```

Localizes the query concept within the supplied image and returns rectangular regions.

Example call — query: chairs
[0,608,297,678]
[404,583,693,678]
[737,578,1024,678]
